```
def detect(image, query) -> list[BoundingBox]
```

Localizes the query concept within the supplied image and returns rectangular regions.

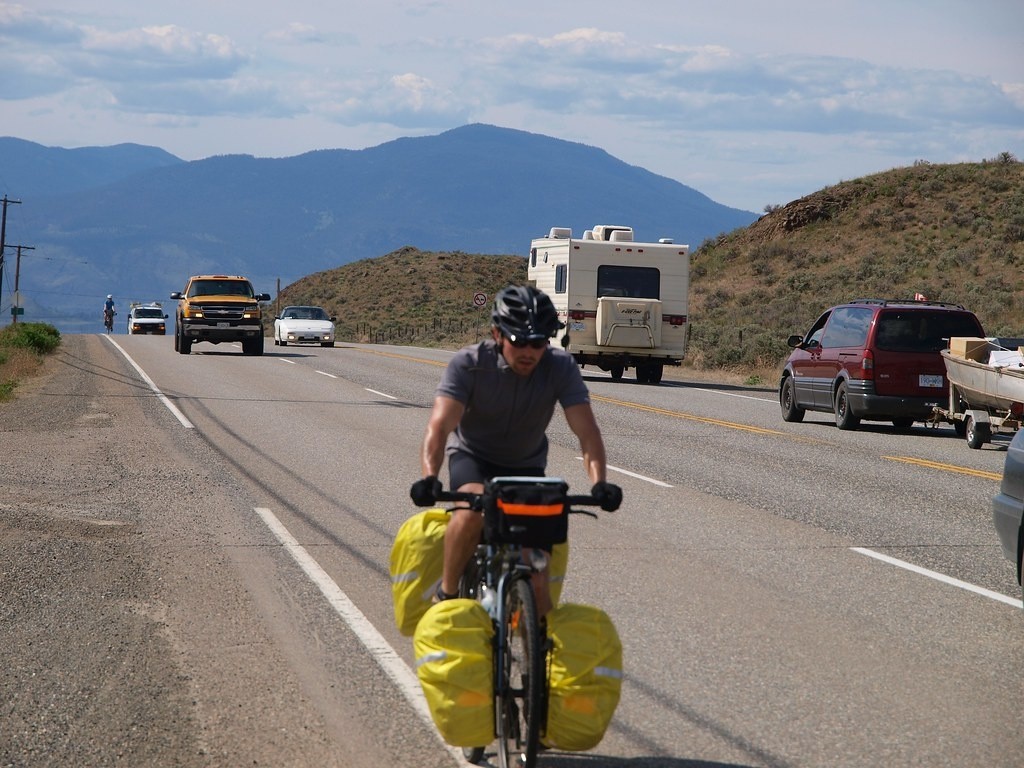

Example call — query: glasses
[499,330,554,349]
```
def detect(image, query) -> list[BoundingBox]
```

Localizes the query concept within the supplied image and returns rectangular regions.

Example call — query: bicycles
[105,310,115,335]
[428,482,614,768]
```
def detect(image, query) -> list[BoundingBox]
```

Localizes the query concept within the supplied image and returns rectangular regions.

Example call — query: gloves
[410,476,442,507]
[104,310,106,312]
[113,311,115,313]
[592,481,623,512]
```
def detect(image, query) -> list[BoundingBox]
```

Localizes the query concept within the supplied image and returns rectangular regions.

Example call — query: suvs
[127,302,169,335]
[170,274,270,355]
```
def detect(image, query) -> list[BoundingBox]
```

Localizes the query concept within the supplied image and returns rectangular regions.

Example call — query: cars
[274,306,337,348]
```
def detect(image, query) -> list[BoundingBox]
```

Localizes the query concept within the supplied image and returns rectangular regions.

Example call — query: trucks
[528,225,690,385]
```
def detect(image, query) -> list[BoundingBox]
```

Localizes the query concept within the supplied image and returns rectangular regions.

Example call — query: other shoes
[111,327,113,331]
[436,579,460,601]
[105,321,107,325]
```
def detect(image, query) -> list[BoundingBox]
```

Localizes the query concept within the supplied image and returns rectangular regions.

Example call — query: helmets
[107,295,112,298]
[491,285,558,340]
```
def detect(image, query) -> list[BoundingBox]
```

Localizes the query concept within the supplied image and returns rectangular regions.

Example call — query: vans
[780,299,984,430]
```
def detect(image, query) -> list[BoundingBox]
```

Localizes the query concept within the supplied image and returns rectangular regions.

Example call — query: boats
[940,339,1024,418]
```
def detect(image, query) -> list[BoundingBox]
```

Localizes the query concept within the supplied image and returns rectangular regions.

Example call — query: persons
[104,295,117,332]
[410,284,623,606]
